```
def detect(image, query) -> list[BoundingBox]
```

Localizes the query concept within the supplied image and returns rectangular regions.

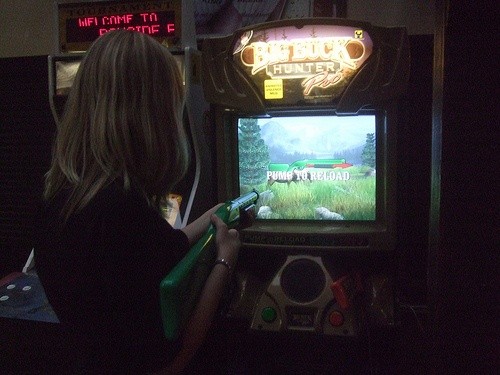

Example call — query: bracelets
[213,258,232,284]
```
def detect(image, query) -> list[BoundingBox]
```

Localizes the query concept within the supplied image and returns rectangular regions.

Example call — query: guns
[160,188,260,342]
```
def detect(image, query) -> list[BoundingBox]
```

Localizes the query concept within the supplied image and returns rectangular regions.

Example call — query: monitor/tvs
[216,106,396,237]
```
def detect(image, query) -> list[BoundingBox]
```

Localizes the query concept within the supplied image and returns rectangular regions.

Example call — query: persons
[34,28,259,375]
